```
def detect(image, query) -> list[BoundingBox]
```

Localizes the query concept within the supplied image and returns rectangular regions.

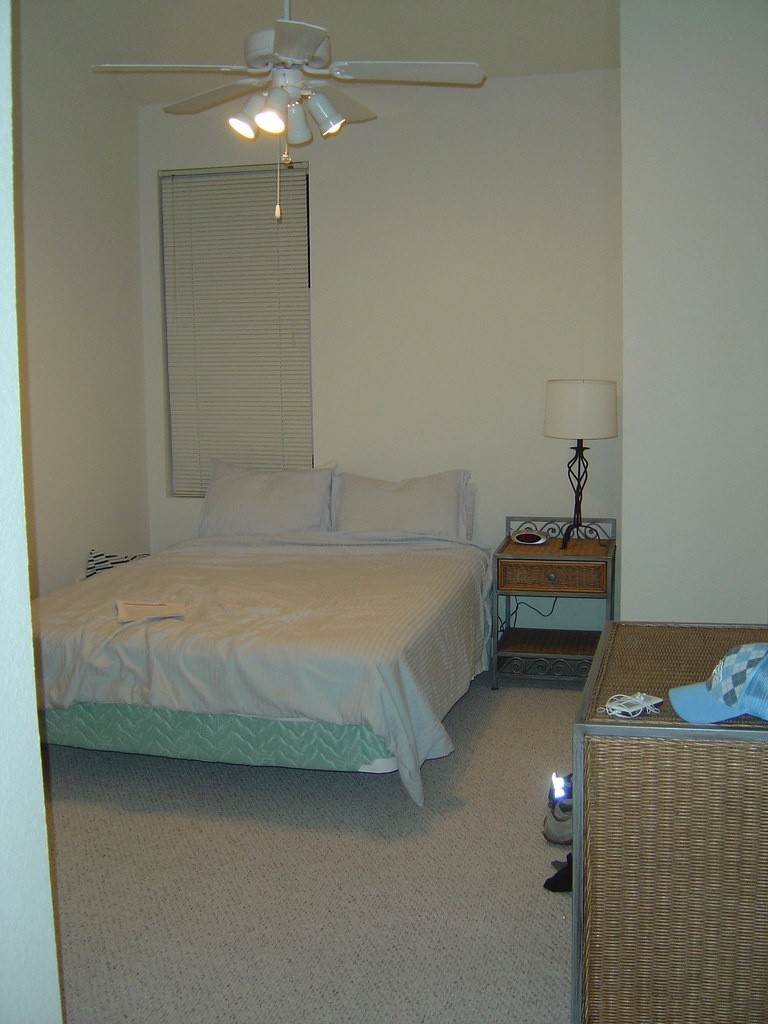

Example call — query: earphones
[637,691,647,698]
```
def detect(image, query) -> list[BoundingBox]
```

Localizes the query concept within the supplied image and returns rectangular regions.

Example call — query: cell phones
[605,694,664,716]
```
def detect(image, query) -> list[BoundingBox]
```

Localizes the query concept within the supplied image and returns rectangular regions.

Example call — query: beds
[31,460,493,807]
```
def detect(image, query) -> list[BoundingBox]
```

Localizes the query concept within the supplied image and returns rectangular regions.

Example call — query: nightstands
[491,516,618,691]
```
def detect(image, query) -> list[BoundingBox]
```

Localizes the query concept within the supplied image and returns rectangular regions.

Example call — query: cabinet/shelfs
[571,620,768,1024]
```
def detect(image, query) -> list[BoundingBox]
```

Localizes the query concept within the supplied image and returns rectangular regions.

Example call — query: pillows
[329,469,471,541]
[199,460,337,538]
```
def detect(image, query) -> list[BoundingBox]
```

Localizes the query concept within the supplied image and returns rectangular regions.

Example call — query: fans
[92,0,484,123]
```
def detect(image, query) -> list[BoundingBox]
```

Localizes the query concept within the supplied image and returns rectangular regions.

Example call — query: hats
[668,642,768,725]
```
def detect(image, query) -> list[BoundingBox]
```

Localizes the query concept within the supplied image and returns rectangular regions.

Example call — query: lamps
[229,85,345,220]
[544,378,617,550]
